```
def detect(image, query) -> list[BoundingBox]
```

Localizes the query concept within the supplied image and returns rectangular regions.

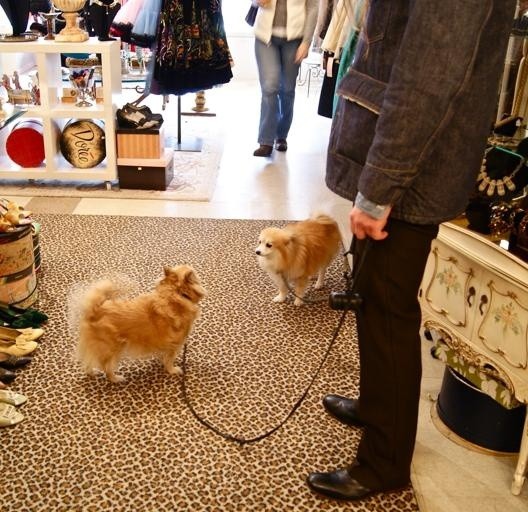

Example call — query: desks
[417,219,528,496]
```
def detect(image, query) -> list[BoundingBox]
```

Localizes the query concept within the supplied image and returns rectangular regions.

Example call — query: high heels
[117,102,164,130]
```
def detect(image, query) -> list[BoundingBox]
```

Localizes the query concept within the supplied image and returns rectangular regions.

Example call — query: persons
[253,1,320,159]
[303,1,522,498]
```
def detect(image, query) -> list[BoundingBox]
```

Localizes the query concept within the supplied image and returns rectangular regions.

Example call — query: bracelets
[509,208,524,236]
[517,214,528,246]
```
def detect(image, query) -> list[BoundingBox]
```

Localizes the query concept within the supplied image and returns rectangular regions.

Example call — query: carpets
[0,214,419,512]
[2,125,225,201]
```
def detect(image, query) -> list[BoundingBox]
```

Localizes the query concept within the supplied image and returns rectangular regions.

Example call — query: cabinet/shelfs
[0,37,122,190]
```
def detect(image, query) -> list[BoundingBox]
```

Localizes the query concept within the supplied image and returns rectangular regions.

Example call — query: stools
[297,59,330,97]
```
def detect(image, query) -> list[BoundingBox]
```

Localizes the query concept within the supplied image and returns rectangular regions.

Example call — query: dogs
[255,211,344,307]
[68,265,208,384]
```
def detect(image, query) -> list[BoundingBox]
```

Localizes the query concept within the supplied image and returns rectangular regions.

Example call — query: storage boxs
[116,128,163,159]
[117,150,175,191]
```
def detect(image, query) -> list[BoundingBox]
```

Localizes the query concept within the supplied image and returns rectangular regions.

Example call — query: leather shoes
[323,395,362,426]
[307,467,407,500]
[253,138,287,157]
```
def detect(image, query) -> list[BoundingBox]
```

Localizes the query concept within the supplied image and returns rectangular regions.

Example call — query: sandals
[0,302,48,426]
[1,198,34,233]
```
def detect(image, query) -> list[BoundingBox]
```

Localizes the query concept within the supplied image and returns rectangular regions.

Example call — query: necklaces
[92,0,118,16]
[477,144,525,196]
